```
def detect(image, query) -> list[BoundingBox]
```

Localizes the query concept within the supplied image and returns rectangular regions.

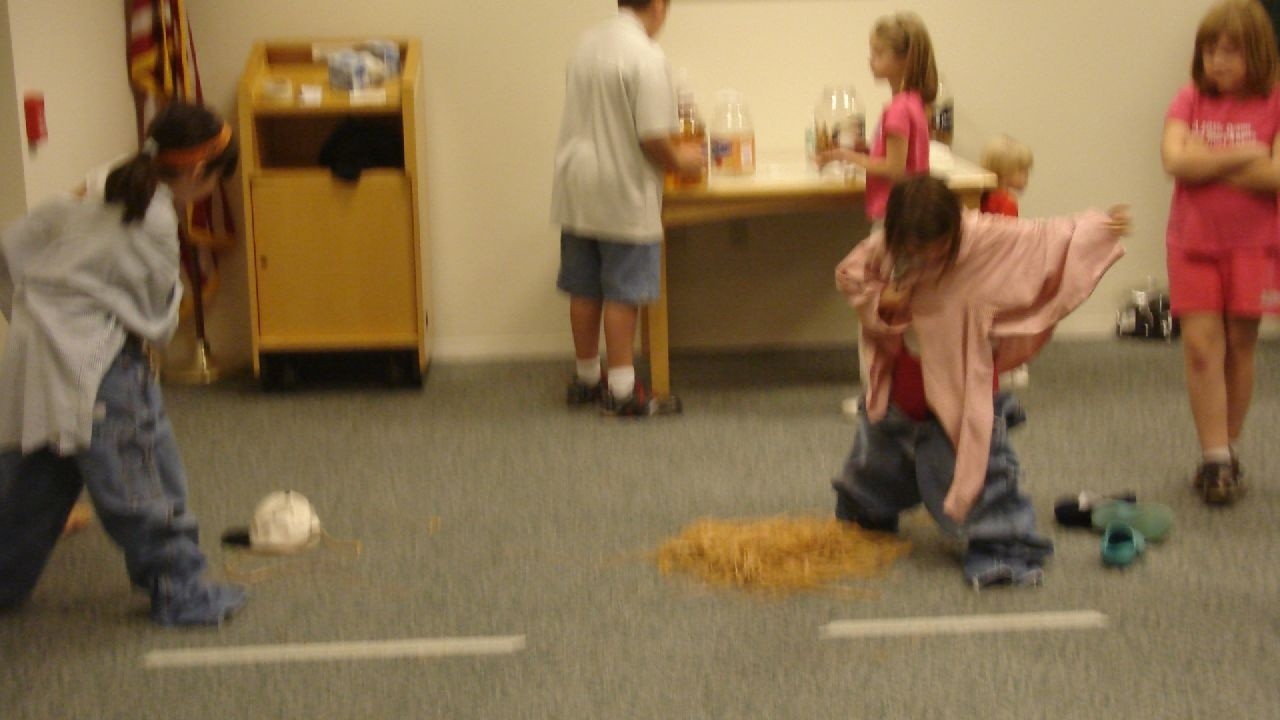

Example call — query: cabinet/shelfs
[237,37,430,382]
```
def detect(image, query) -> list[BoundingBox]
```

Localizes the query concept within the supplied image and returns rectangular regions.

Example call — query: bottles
[710,91,756,176]
[814,86,866,153]
[667,91,709,190]
[925,79,953,146]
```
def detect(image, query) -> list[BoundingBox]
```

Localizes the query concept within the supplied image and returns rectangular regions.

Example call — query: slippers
[1090,503,1175,538]
[1100,519,1147,566]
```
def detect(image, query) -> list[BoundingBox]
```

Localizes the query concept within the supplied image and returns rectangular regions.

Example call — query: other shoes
[601,380,683,416]
[842,395,861,421]
[1053,495,1139,524]
[1193,446,1242,503]
[173,582,251,624]
[567,376,608,407]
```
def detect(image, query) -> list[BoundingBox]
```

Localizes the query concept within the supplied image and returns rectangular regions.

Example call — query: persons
[1161,0,1280,508]
[0,102,248,628]
[816,9,938,421]
[548,0,703,420]
[830,175,1135,592]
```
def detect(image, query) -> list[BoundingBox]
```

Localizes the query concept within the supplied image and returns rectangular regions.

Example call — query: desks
[642,153,997,394]
